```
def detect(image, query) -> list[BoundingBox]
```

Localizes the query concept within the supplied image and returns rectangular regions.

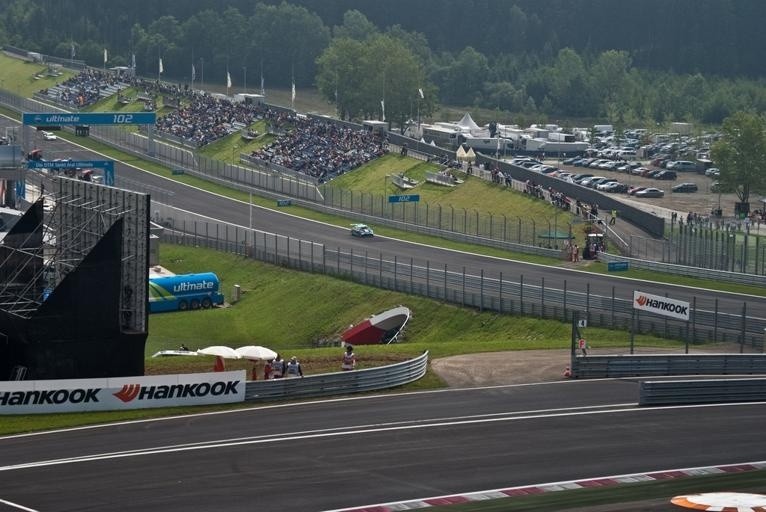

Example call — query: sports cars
[352,223,373,237]
[42,132,56,141]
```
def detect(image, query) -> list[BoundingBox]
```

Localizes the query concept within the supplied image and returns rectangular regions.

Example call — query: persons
[340,345,356,371]
[272,354,285,379]
[133,78,256,146]
[427,155,625,263]
[282,356,305,378]
[400,143,408,156]
[254,109,388,183]
[40,66,132,108]
[664,201,766,235]
[264,359,273,378]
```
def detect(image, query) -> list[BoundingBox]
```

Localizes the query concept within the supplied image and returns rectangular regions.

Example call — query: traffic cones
[564,367,569,375]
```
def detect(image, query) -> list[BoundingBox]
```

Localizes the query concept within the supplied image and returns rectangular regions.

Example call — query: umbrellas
[229,344,277,378]
[191,343,241,371]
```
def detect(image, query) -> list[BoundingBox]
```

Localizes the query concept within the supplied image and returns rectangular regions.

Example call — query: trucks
[148,271,224,313]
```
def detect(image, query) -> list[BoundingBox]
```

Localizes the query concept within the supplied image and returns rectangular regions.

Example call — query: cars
[513,129,734,197]
